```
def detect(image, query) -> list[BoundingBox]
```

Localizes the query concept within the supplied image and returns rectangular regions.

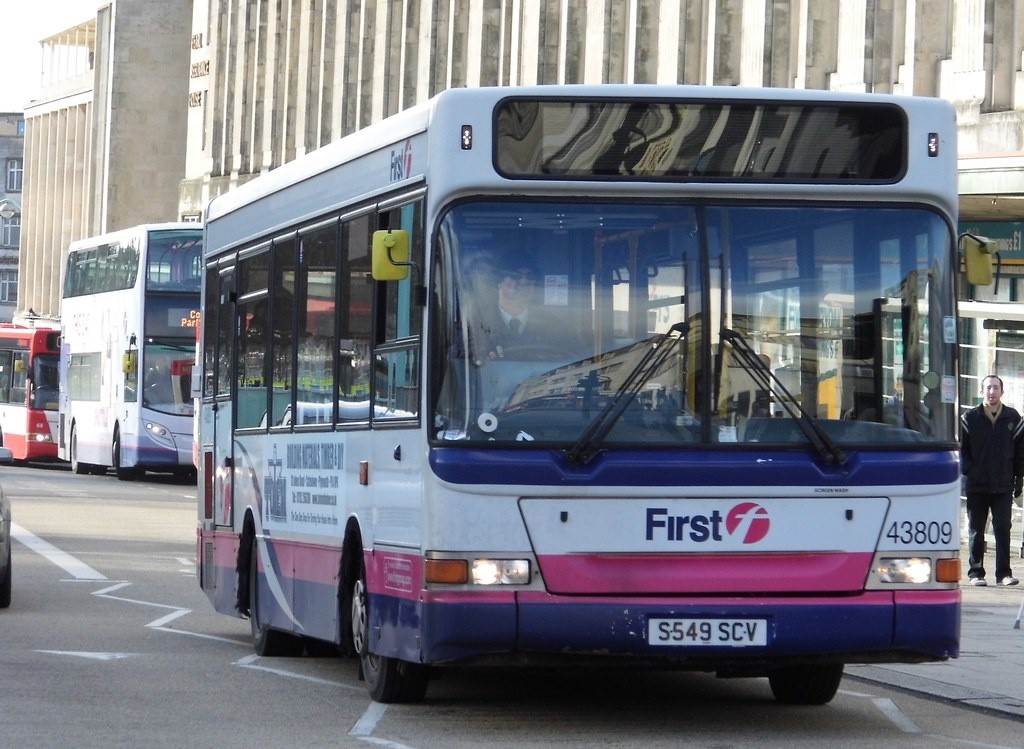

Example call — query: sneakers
[996,575,1019,585]
[970,577,987,586]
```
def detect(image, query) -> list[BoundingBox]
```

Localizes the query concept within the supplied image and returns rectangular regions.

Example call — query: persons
[955,373,1024,588]
[145,353,172,399]
[442,247,576,432]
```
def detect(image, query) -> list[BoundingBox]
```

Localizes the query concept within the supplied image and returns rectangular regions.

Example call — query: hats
[494,253,540,273]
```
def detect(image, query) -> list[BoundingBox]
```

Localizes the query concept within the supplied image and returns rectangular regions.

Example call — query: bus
[0,322,61,464]
[58,221,204,484]
[197,85,1003,704]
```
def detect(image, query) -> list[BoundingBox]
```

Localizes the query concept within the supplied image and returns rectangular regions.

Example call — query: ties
[508,319,522,343]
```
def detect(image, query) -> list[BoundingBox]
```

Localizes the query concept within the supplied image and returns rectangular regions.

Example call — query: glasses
[507,271,537,281]
